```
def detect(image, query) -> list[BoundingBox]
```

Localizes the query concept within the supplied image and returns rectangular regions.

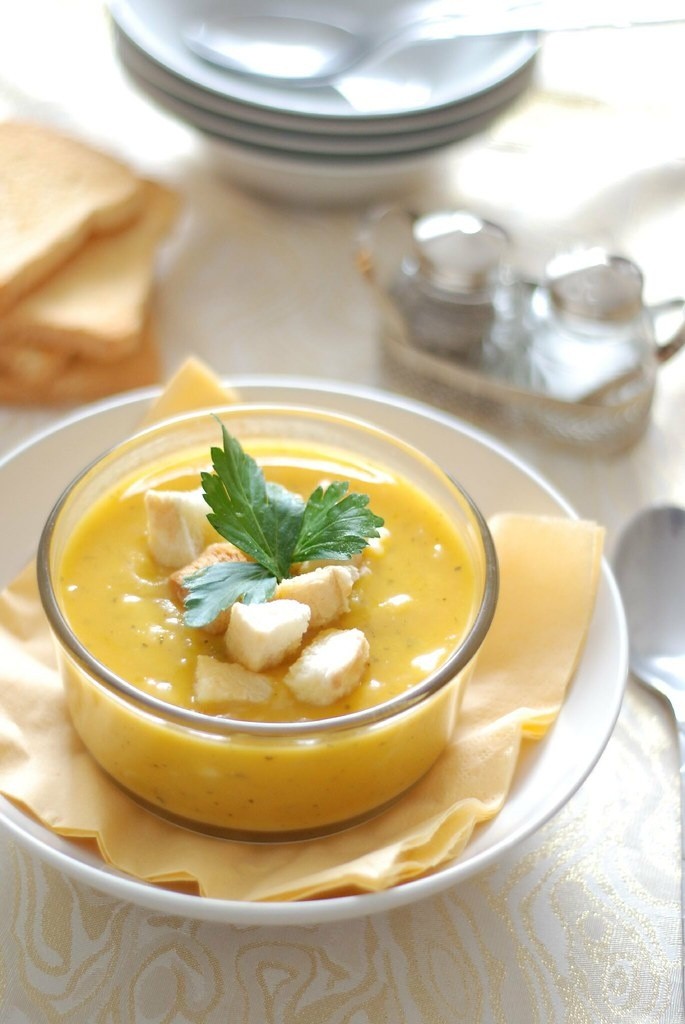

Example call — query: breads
[0,116,183,411]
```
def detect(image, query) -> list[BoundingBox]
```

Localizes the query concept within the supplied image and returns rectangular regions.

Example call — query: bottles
[397,209,655,406]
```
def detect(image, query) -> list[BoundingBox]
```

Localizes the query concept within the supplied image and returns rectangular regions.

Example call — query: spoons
[184,1,685,89]
[611,505,685,1022]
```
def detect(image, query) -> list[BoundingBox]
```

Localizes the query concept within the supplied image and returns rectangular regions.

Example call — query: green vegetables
[179,414,384,627]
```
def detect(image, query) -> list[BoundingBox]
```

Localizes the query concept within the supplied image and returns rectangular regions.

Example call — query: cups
[36,404,501,849]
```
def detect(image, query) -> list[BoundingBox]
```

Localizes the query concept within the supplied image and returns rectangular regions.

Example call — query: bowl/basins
[1,376,630,928]
[103,1,542,207]
